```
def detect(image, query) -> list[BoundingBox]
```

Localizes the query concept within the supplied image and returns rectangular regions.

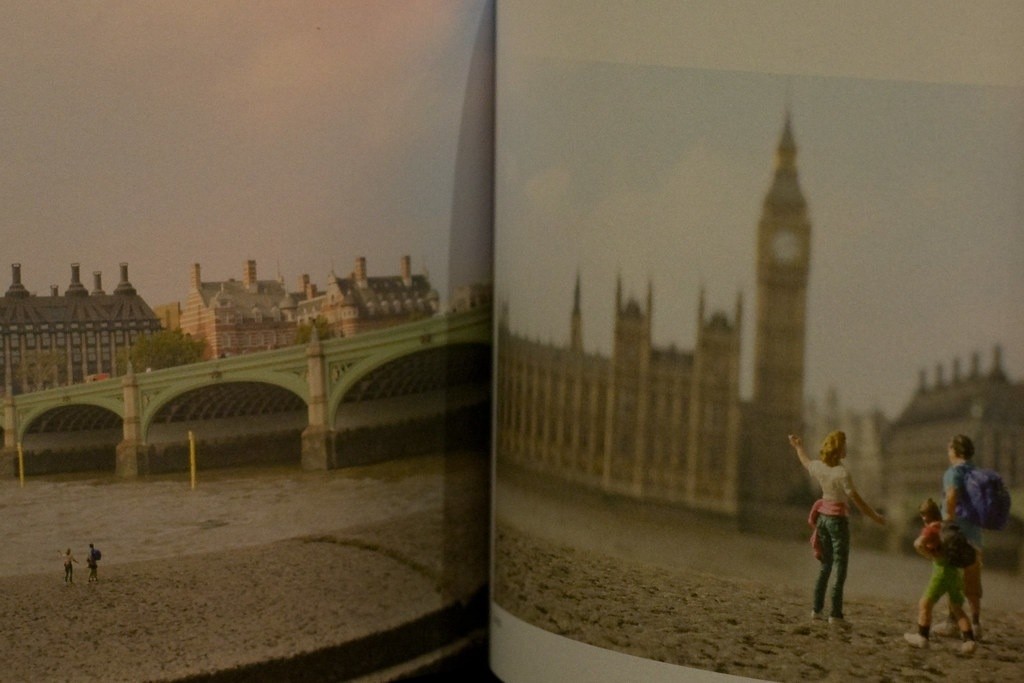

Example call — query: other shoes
[65,578,68,583]
[971,624,981,641]
[812,610,828,621]
[904,632,928,650]
[69,579,73,583]
[829,616,853,628]
[932,621,958,637]
[962,640,976,654]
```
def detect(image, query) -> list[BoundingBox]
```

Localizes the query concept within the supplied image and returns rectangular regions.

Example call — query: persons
[87,544,98,582]
[932,435,981,639]
[57,548,77,584]
[904,501,976,654]
[787,431,889,625]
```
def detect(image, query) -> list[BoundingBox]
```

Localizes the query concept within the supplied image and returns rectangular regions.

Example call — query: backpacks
[955,466,1011,529]
[937,521,975,566]
[91,549,101,561]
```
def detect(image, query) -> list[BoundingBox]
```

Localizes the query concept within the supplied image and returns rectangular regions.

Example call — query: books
[0,0,1024,683]
